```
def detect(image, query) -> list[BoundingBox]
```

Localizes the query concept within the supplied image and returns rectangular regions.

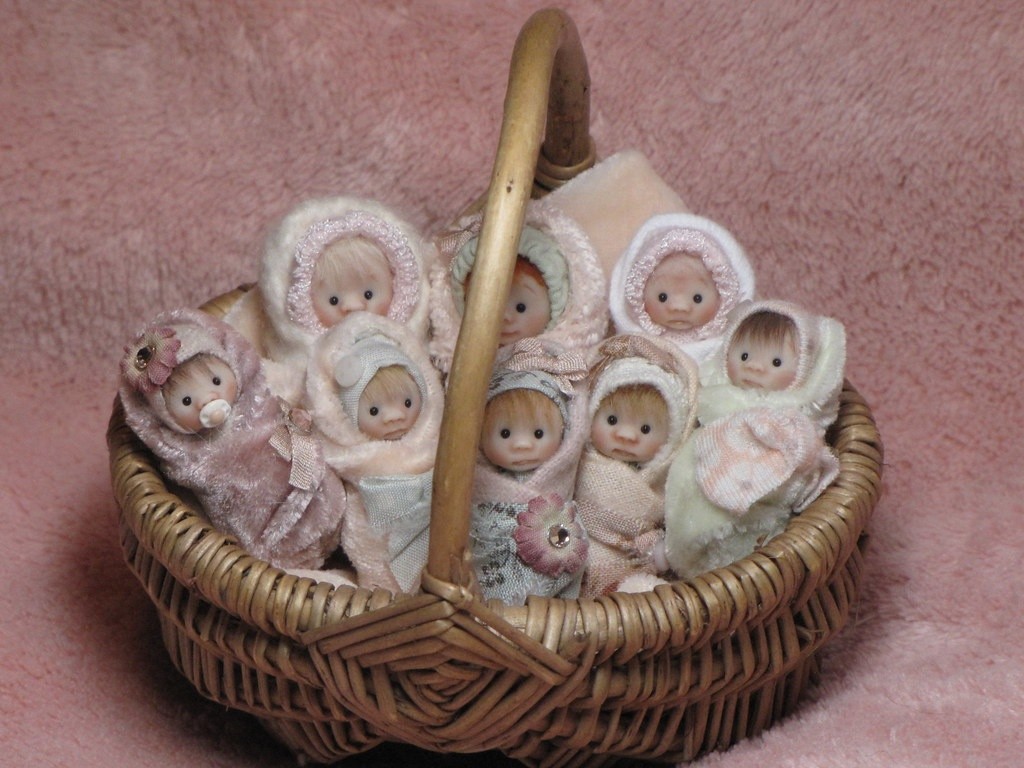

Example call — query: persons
[263,196,440,410]
[568,331,703,597]
[462,339,591,600]
[659,299,846,581]
[301,311,443,594]
[425,199,609,374]
[116,306,345,570]
[609,213,756,378]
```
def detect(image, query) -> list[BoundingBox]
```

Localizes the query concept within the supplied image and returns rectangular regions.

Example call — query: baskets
[106,7,885,768]
